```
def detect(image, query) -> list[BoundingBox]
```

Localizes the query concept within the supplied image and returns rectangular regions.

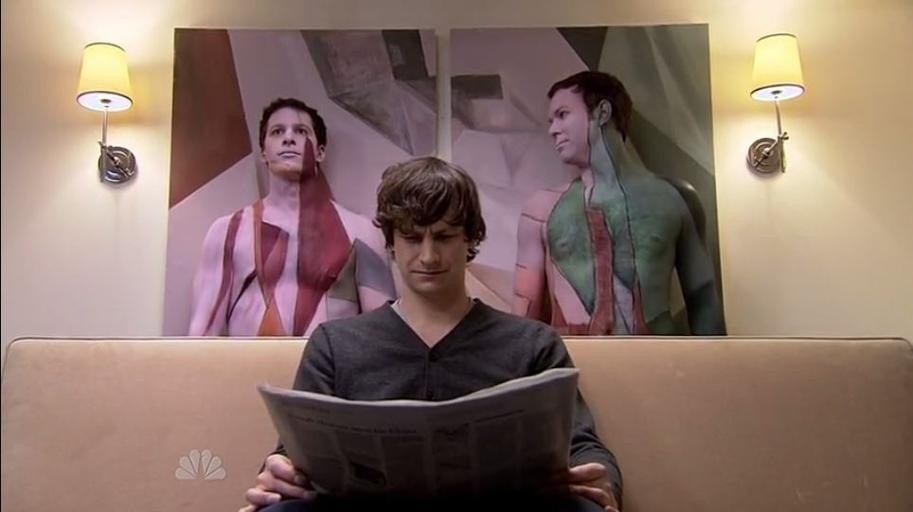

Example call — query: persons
[187,97,398,337]
[235,155,623,512]
[510,67,727,337]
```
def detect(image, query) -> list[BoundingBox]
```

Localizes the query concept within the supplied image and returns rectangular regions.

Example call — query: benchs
[6,336,910,511]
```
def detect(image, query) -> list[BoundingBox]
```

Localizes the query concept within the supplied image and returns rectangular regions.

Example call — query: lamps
[746,33,805,175]
[75,42,142,185]
